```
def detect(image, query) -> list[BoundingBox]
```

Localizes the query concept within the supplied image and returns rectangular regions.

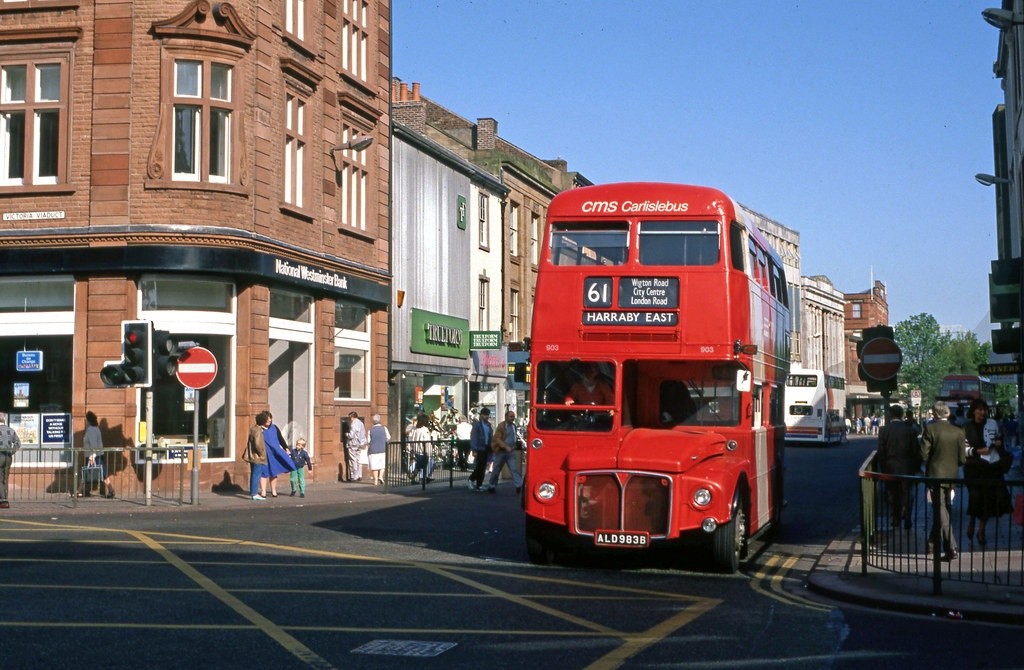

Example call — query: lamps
[330,136,374,154]
[975,172,1012,186]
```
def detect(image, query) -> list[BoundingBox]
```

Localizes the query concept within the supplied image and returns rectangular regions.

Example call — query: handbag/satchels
[82,460,103,482]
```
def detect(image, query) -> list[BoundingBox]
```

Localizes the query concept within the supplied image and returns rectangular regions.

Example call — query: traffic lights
[100,318,153,390]
[150,328,179,382]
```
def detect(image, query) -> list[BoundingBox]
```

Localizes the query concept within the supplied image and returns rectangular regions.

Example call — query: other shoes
[290,491,296,496]
[488,487,496,493]
[300,494,304,498]
[476,486,486,491]
[467,478,473,490]
[272,492,277,497]
[967,522,974,539]
[904,521,913,529]
[927,542,934,554]
[941,549,958,562]
[260,491,266,497]
[976,533,987,544]
[378,477,383,484]
[891,520,901,528]
[516,487,521,493]
[252,496,266,500]
[0,502,9,508]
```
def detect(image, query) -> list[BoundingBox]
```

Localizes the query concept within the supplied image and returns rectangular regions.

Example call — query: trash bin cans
[501,441,522,480]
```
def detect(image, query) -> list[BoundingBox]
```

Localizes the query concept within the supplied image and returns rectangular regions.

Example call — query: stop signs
[177,346,218,390]
[860,337,901,379]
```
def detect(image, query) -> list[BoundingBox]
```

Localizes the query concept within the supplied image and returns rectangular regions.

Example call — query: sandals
[72,492,83,496]
[107,491,115,498]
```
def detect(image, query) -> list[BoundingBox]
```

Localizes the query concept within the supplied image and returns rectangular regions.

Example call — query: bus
[521,180,791,573]
[939,375,999,422]
[783,369,848,445]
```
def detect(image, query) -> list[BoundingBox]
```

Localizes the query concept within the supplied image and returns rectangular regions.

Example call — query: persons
[927,415,960,505]
[905,411,916,424]
[78,411,115,498]
[992,413,1019,435]
[466,408,493,491]
[845,416,852,433]
[406,414,435,484]
[852,415,881,436]
[923,409,934,428]
[0,413,20,508]
[247,411,296,501]
[368,415,391,485]
[288,438,312,498]
[879,405,922,529]
[564,363,614,416]
[961,399,1013,545]
[452,415,473,471]
[488,411,523,493]
[921,401,965,559]
[344,412,367,481]
